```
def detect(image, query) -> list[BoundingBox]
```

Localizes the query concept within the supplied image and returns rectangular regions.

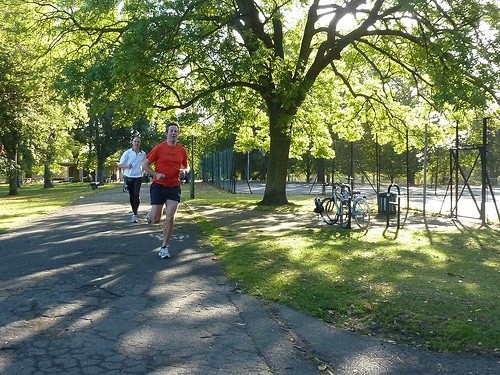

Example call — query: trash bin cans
[380,192,397,214]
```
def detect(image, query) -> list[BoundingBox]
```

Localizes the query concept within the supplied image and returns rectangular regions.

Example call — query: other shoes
[123,188,126,192]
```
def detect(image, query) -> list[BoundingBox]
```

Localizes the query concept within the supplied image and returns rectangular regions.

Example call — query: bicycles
[319,177,371,230]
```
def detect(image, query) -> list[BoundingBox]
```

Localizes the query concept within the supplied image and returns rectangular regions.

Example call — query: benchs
[90,181,100,190]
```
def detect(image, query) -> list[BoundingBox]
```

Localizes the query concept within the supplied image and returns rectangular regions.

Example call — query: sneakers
[147,213,152,224]
[159,248,170,259]
[132,215,137,223]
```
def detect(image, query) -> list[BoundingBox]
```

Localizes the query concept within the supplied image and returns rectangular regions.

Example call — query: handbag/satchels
[314,198,324,213]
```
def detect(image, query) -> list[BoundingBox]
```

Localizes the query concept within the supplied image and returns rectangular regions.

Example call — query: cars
[179,164,192,184]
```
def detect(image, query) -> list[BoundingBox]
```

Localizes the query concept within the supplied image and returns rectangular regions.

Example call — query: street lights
[422,116,429,218]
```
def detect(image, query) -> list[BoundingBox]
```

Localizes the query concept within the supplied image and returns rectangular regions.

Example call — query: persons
[180,169,191,184]
[141,123,191,260]
[122,180,128,192]
[117,136,146,224]
